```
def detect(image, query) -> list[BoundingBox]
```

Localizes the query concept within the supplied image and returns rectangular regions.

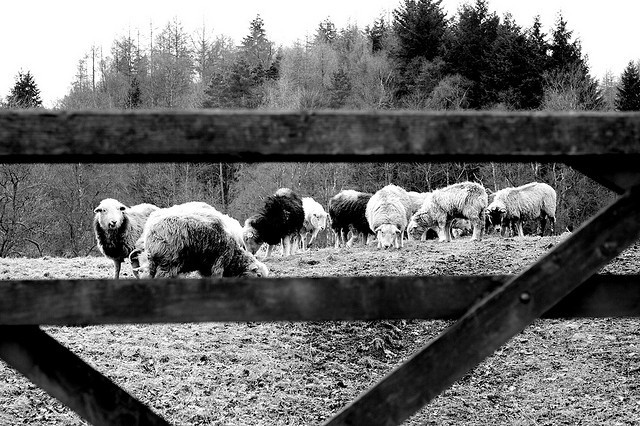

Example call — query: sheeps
[406,179,487,243]
[366,183,411,249]
[299,197,328,250]
[409,192,469,242]
[135,209,268,279]
[329,189,376,249]
[486,182,559,235]
[174,199,252,250]
[245,186,305,257]
[94,196,160,279]
